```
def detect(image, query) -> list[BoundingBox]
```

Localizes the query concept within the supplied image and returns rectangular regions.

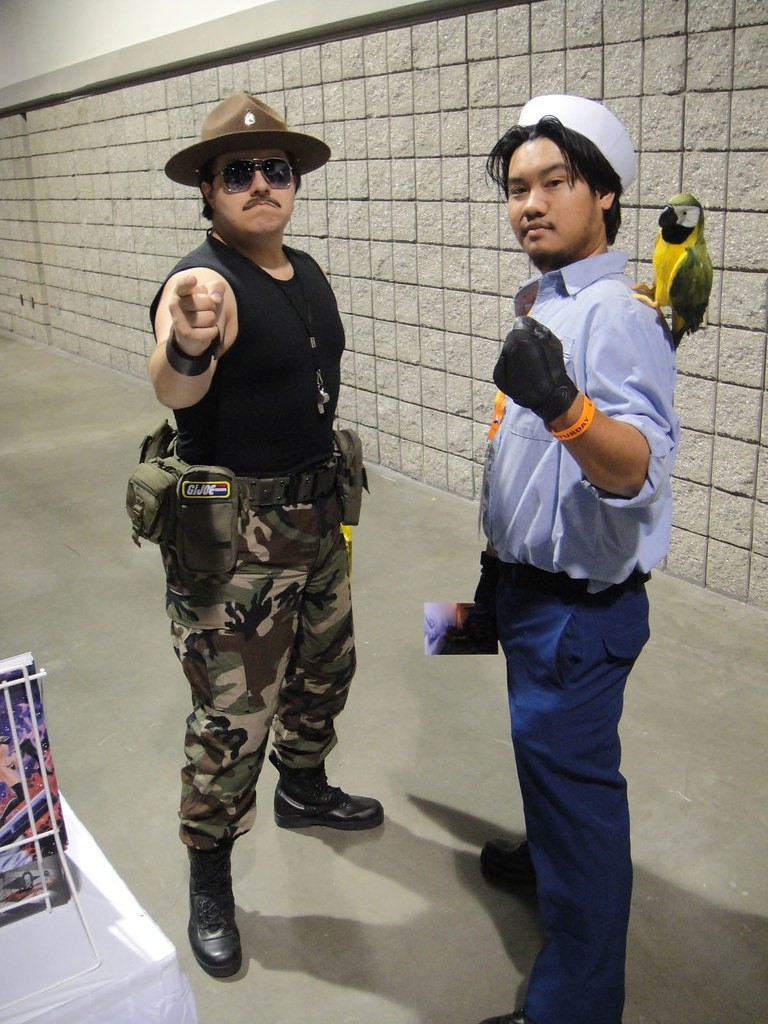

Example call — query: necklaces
[216,230,330,416]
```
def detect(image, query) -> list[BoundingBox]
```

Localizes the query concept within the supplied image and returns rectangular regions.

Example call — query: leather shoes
[478,1007,539,1024]
[479,837,538,901]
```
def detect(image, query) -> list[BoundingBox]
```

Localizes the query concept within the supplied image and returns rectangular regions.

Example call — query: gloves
[493,314,578,425]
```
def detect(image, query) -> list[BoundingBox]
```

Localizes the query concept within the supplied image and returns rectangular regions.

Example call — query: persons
[464,95,681,1024]
[149,92,384,978]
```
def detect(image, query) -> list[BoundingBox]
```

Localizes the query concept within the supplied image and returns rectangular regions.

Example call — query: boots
[186,840,242,977]
[268,748,384,831]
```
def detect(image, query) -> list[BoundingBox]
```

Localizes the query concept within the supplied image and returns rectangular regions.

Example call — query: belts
[172,439,340,506]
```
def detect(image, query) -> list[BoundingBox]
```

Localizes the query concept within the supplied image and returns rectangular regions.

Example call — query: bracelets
[543,392,595,440]
[166,325,220,376]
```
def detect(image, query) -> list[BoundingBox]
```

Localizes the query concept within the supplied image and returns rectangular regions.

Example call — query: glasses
[211,155,293,195]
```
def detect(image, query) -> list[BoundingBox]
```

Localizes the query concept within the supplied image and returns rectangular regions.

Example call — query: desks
[0,788,198,1024]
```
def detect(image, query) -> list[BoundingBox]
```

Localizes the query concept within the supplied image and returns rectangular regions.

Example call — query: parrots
[630,193,713,352]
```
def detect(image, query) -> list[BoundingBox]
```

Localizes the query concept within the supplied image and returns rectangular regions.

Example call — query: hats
[163,92,332,187]
[516,94,637,194]
